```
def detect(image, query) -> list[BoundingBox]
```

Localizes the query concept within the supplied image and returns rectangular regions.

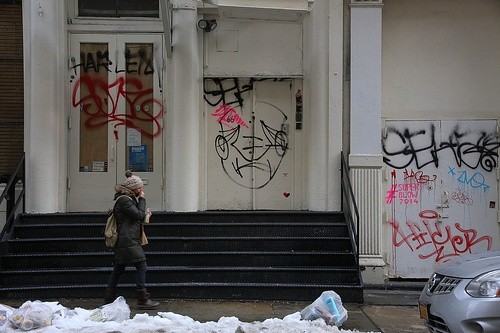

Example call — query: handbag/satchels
[105,196,137,248]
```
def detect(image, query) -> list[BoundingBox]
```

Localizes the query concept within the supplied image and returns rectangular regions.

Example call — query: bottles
[315,296,342,326]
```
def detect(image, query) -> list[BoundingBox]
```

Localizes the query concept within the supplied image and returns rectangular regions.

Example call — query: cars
[417,249,500,333]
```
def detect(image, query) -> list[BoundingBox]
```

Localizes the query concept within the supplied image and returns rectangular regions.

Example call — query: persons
[104,170,160,309]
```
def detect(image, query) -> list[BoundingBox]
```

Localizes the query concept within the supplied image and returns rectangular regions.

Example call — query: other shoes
[137,298,160,309]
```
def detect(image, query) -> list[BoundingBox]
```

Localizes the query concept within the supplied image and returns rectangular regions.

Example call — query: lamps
[197,19,217,32]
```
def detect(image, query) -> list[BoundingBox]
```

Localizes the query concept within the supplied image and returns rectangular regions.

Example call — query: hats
[124,170,144,190]
